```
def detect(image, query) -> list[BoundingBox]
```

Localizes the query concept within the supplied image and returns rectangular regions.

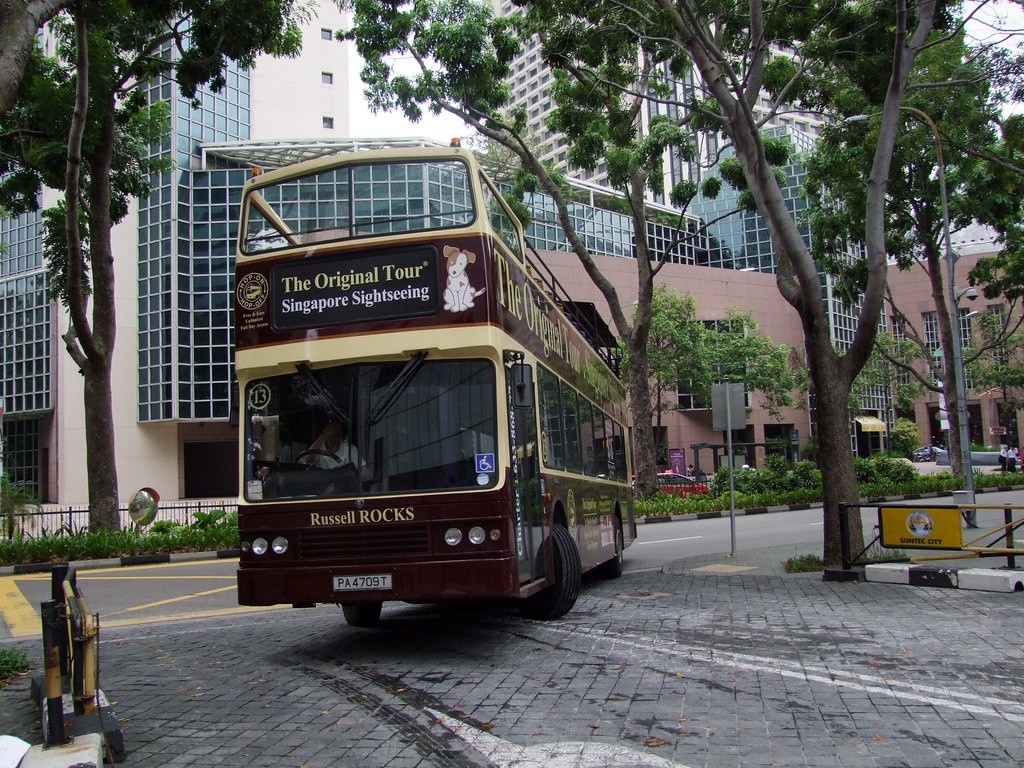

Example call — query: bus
[235,137,638,627]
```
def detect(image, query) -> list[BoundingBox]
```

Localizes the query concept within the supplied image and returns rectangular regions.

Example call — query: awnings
[854,416,886,432]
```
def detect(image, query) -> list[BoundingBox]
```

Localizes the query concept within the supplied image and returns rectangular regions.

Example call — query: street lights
[843,107,979,528]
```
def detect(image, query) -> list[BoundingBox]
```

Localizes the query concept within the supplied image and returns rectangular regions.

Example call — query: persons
[928,444,933,457]
[1000,445,1008,470]
[1008,446,1016,471]
[1014,447,1024,472]
[305,415,368,477]
[688,465,693,476]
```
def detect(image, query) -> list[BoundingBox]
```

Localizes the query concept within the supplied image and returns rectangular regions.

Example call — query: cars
[660,473,710,499]
